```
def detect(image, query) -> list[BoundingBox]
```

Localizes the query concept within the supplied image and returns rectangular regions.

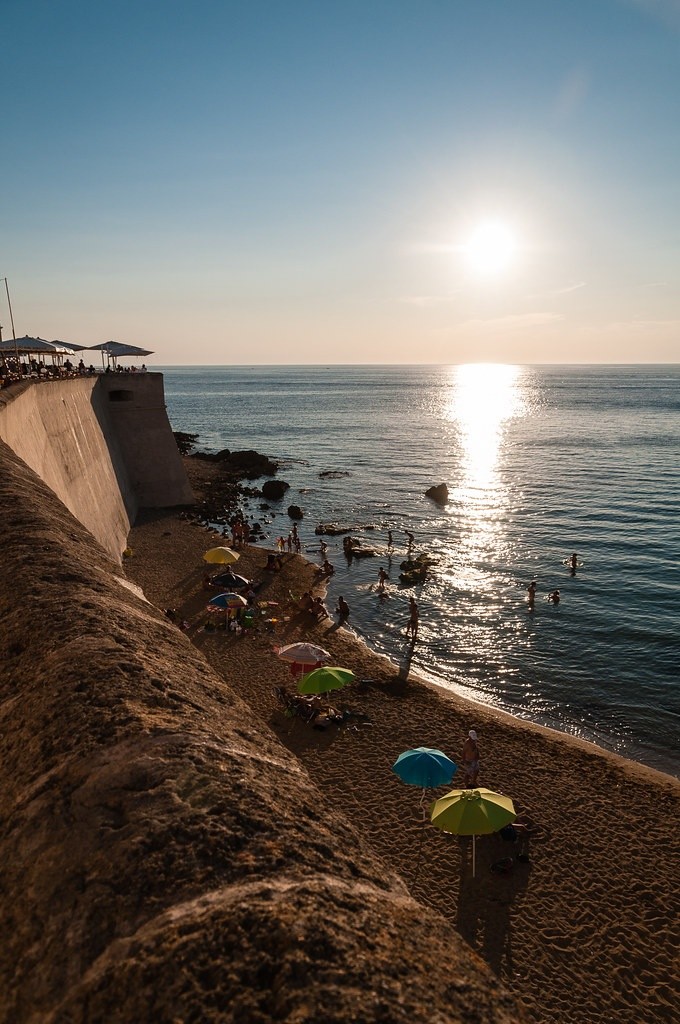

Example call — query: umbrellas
[393,746,518,878]
[0,334,153,370]
[277,641,356,702]
[211,573,249,592]
[203,546,241,564]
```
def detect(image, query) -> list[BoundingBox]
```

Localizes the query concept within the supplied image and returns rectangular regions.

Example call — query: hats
[468,730,477,739]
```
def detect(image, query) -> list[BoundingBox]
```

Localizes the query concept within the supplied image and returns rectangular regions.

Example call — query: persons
[272,555,334,574]
[528,553,579,604]
[299,589,349,617]
[398,561,428,585]
[277,522,301,554]
[280,686,323,711]
[405,596,420,638]
[405,530,416,550]
[460,729,537,834]
[162,609,179,619]
[0,357,96,381]
[105,364,148,373]
[378,566,387,587]
[204,574,213,581]
[387,531,394,552]
[231,519,251,547]
[343,536,354,548]
[223,565,233,573]
[318,539,327,552]
[209,594,248,630]
[314,703,341,726]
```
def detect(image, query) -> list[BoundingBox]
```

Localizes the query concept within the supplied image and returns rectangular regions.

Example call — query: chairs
[274,686,316,725]
